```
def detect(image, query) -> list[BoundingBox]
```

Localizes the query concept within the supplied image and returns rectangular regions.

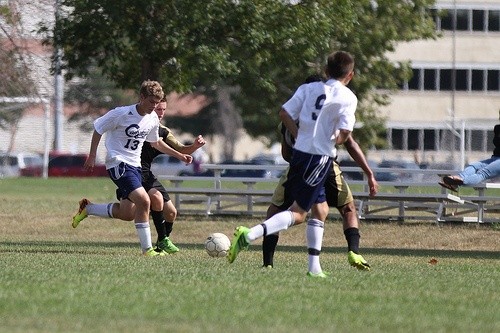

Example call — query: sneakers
[72,197,91,229]
[347,251,372,271]
[306,271,329,281]
[142,246,165,258]
[226,226,251,265]
[154,237,180,254]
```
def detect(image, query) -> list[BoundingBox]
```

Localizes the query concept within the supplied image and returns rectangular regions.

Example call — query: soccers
[205,232,230,257]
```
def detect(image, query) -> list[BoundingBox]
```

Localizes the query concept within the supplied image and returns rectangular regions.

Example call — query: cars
[18,152,108,177]
[188,160,276,178]
[0,152,44,175]
[251,160,436,185]
[144,145,203,178]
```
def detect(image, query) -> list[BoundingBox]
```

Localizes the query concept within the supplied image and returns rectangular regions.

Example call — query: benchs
[154,160,500,227]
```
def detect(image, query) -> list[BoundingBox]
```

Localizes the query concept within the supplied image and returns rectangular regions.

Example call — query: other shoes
[443,175,463,186]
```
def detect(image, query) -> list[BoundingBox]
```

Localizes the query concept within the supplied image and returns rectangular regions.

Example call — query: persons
[443,110,500,186]
[72,79,193,256]
[226,51,357,281]
[115,92,207,253]
[262,74,379,270]
